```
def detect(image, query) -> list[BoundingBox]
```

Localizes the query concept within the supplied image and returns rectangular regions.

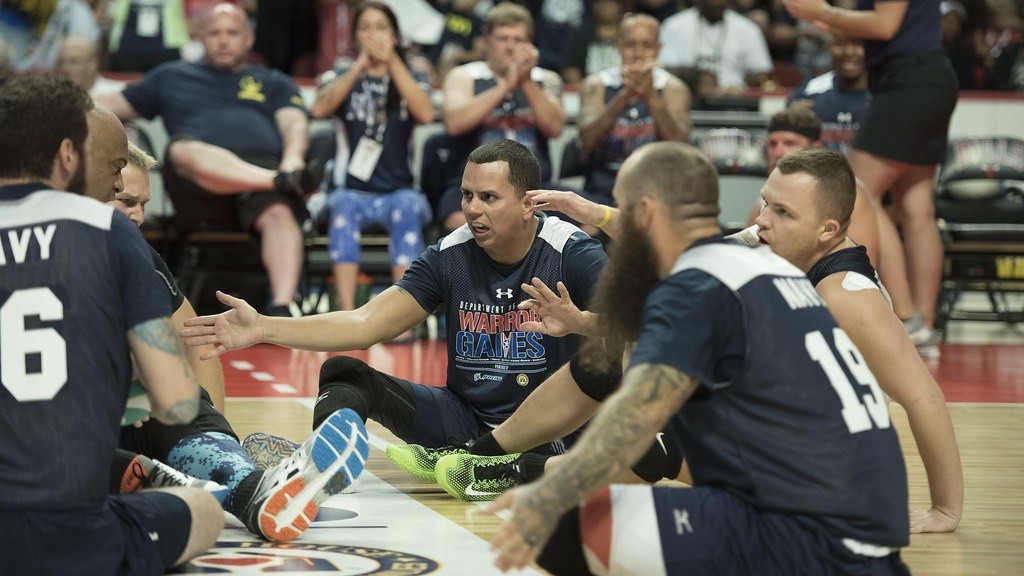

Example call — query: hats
[770,108,822,141]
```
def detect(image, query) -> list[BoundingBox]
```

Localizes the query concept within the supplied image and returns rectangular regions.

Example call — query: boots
[239,382,370,495]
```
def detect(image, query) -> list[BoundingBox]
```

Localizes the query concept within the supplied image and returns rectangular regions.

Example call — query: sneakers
[386,436,479,482]
[240,408,363,543]
[435,450,530,501]
[118,455,229,512]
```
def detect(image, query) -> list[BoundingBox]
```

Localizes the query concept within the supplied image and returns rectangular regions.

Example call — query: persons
[0,0,1024,576]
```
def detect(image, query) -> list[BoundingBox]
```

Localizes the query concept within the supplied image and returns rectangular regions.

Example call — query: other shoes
[278,157,324,204]
[903,313,944,362]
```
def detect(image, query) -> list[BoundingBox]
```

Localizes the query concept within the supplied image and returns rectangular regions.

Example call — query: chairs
[412,124,459,244]
[933,136,1024,345]
[547,125,586,198]
[293,120,336,196]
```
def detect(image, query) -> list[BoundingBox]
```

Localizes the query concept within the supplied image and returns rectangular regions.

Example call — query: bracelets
[594,204,610,228]
